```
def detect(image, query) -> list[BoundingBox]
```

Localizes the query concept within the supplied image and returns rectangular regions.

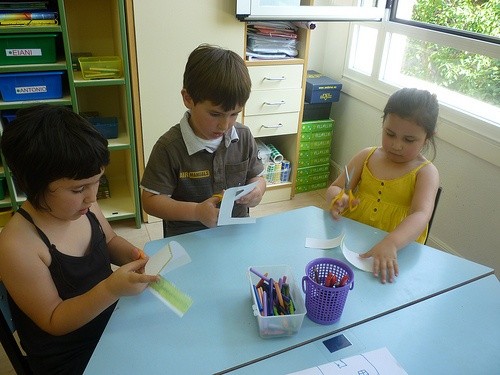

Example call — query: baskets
[305,257,355,325]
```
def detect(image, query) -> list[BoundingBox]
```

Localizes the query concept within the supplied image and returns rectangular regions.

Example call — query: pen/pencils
[250,267,298,337]
[314,269,349,288]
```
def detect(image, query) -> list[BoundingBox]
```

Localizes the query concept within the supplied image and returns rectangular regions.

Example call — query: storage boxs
[248,258,355,339]
[0,71,63,102]
[78,56,123,80]
[257,168,291,185]
[82,117,119,141]
[294,70,343,194]
[0,34,57,66]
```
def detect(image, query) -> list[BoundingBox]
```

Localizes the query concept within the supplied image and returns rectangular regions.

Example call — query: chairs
[0,278,35,375]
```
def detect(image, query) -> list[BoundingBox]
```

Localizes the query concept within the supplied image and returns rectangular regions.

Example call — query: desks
[83,205,500,375]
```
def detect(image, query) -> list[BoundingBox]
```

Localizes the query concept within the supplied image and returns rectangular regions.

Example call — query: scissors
[330,165,357,214]
[212,189,244,204]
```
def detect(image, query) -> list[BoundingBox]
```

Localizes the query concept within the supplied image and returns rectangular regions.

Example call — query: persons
[0,105,162,375]
[324,87,441,285]
[139,43,267,239]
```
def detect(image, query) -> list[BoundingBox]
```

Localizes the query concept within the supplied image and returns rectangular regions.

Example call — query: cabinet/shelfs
[0,0,310,228]
